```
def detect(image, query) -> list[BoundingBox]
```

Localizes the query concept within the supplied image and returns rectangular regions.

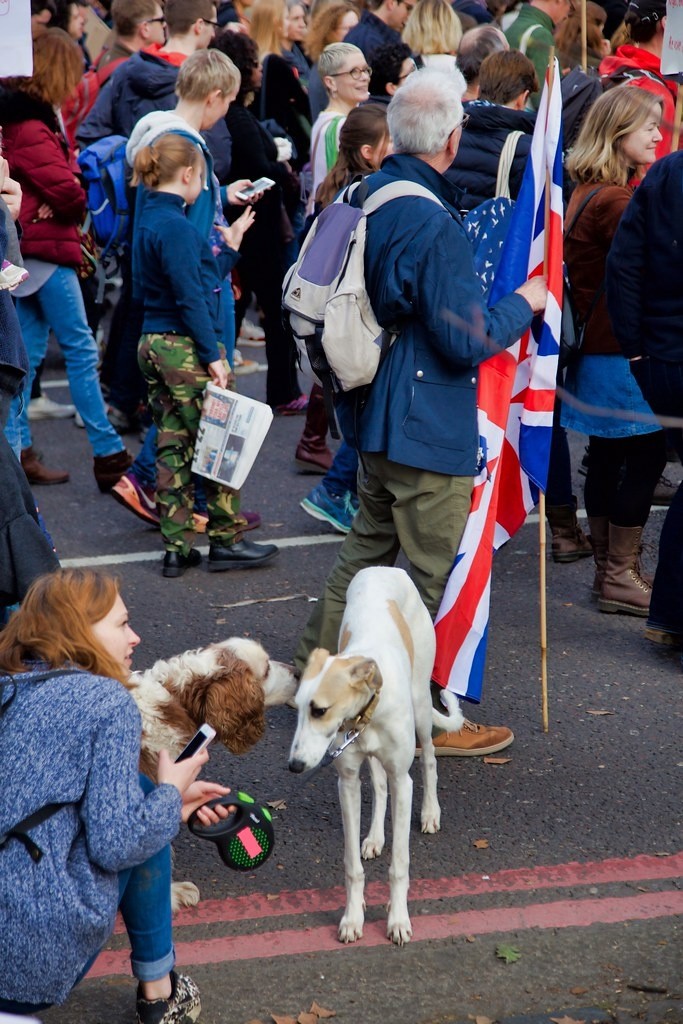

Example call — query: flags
[426,56,567,706]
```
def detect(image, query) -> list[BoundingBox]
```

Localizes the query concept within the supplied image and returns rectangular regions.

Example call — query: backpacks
[62,48,129,150]
[559,66,662,149]
[281,176,446,395]
[74,134,136,259]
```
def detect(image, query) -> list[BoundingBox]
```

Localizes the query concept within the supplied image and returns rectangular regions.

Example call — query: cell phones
[234,176,276,201]
[174,723,215,765]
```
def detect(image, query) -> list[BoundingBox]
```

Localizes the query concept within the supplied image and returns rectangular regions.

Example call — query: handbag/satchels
[465,131,571,308]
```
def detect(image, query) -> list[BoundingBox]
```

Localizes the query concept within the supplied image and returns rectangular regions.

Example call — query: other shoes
[295,434,334,468]
[646,627,683,647]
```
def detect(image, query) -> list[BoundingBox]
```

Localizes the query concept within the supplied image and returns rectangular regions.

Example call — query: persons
[0,0,683,753]
[0,555,233,1023]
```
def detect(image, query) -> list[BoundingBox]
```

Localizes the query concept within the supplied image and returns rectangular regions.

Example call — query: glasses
[402,0,415,11]
[145,14,164,24]
[189,19,223,34]
[329,65,373,80]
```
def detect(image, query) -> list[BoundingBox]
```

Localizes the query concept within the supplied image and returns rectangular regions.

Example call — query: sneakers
[236,317,263,348]
[192,512,262,533]
[268,392,311,414]
[111,475,161,524]
[415,717,515,756]
[299,484,355,533]
[25,392,76,420]
[136,970,200,1024]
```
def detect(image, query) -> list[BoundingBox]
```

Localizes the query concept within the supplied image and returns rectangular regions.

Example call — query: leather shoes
[163,549,201,577]
[208,538,278,569]
[18,447,69,482]
[93,449,135,492]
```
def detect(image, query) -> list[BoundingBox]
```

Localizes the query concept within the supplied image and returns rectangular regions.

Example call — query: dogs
[128,634,298,793]
[286,566,435,948]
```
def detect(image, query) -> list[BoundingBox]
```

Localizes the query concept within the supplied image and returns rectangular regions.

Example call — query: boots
[545,495,594,563]
[590,519,656,605]
[599,523,653,616]
